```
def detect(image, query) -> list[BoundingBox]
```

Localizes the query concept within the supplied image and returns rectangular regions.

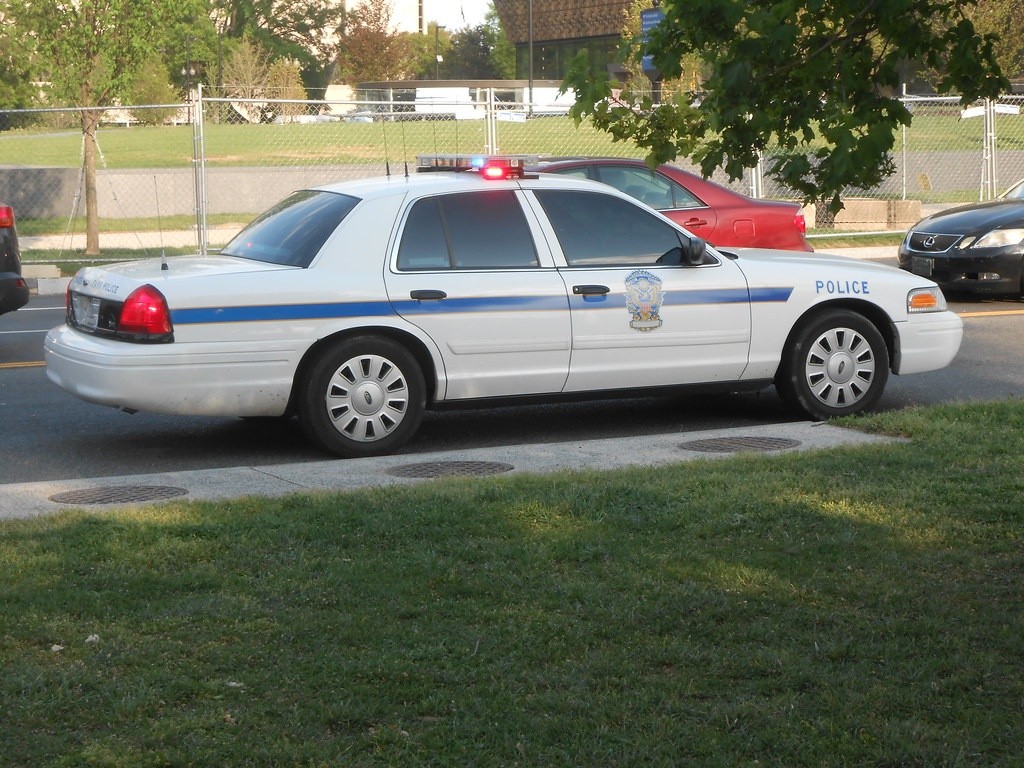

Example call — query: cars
[523,158,818,254]
[897,180,1024,304]
[43,156,965,459]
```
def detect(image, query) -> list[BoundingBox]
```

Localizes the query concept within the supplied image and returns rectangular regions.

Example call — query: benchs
[627,184,685,210]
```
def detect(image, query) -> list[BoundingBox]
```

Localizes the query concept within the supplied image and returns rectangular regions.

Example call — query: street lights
[434,26,446,80]
